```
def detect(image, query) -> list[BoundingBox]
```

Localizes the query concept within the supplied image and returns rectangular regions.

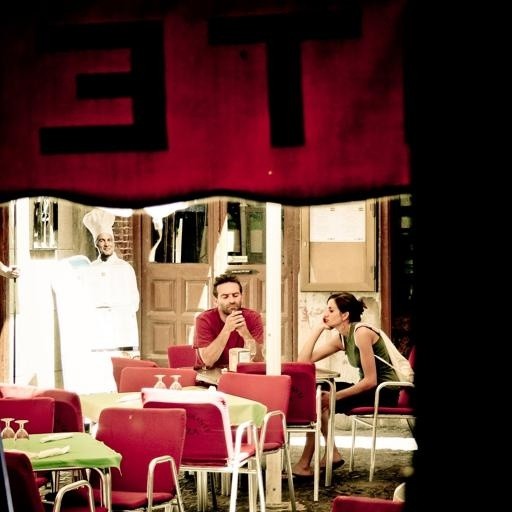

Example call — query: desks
[1,427,125,512]
[109,383,269,450]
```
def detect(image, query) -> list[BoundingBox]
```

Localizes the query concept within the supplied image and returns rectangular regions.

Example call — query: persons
[281,291,415,481]
[192,275,264,372]
[0,261,21,280]
[82,207,140,359]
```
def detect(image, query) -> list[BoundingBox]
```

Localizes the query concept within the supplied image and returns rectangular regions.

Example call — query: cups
[244,339,257,362]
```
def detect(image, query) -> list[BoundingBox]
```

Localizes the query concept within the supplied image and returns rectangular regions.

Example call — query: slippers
[318,459,345,476]
[282,472,313,481]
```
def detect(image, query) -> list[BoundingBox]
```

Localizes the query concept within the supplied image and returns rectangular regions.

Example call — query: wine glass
[0,417,16,450]
[168,375,183,390]
[153,374,167,389]
[14,419,29,451]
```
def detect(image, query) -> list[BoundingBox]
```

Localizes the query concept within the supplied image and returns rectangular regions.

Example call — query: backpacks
[353,321,415,384]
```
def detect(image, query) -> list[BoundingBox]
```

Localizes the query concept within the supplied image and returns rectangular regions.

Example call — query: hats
[82,207,116,242]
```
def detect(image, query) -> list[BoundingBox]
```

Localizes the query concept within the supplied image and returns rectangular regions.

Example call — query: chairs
[110,355,158,386]
[233,361,338,502]
[1,395,57,436]
[331,481,407,511]
[347,345,416,482]
[30,387,86,432]
[2,449,97,512]
[211,371,298,511]
[118,365,201,391]
[80,406,187,511]
[165,346,201,368]
[137,400,269,511]
